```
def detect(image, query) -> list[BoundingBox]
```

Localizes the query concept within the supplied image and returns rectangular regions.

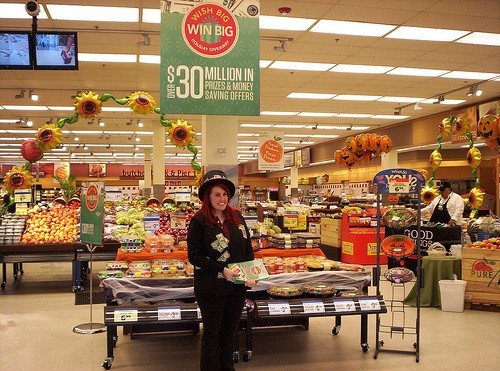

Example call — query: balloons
[478,113,500,149]
[334,132,393,168]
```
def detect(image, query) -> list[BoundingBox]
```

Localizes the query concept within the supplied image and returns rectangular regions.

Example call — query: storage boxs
[321,218,342,248]
[438,279,467,312]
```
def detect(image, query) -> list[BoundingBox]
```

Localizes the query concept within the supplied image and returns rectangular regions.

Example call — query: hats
[198,170,235,202]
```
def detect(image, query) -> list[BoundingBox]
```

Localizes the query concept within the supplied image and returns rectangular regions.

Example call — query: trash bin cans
[439,279,468,311]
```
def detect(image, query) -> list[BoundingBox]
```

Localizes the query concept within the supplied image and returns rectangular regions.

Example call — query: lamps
[433,95,446,104]
[466,84,483,97]
[312,124,317,130]
[14,89,142,156]
[347,125,352,131]
[136,36,151,47]
[394,108,402,115]
[273,41,287,51]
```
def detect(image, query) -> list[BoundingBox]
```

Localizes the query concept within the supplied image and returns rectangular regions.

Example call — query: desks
[403,256,461,308]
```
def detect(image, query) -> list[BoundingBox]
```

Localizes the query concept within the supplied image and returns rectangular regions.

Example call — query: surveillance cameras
[25,0,40,16]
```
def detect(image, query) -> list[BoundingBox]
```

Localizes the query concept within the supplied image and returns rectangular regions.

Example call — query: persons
[412,181,465,226]
[3,195,10,206]
[54,191,59,199]
[186,170,253,371]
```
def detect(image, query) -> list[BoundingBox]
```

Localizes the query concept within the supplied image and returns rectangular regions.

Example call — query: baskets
[426,242,446,257]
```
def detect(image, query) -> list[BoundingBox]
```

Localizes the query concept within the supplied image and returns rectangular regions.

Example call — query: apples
[21,201,80,243]
[154,207,196,241]
[467,237,500,250]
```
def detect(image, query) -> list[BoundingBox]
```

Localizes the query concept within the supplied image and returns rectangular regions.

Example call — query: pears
[115,208,147,237]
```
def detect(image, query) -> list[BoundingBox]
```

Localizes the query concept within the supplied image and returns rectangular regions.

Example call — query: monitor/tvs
[1,31,33,68]
[33,31,79,69]
